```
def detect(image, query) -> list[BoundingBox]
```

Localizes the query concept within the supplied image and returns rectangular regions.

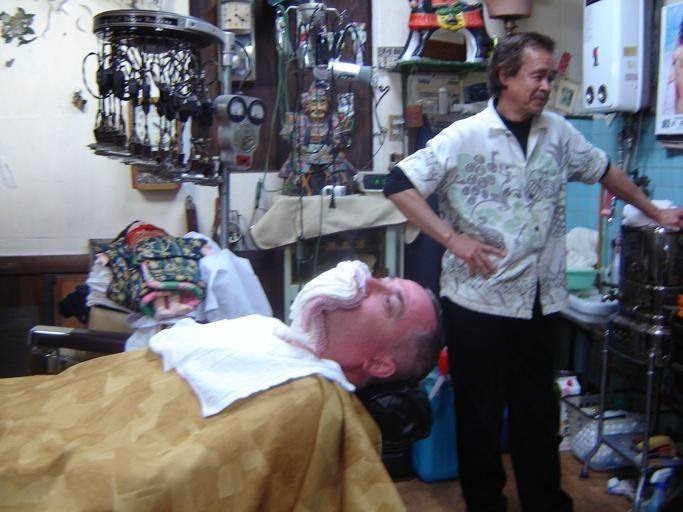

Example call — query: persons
[301,274,443,392]
[383,30,682,511]
[278,81,358,199]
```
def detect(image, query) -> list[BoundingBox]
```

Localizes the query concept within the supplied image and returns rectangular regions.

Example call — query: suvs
[618,222,683,324]
[412,345,459,482]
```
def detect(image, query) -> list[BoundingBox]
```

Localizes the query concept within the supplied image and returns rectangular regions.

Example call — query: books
[414,381,508,482]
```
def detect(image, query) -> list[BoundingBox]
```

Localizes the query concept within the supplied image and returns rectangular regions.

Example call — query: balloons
[125,94,182,191]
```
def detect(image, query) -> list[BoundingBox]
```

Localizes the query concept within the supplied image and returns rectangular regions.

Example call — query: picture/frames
[268,191,406,331]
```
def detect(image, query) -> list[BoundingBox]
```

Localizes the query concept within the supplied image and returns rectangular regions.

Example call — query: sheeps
[600,290,617,303]
